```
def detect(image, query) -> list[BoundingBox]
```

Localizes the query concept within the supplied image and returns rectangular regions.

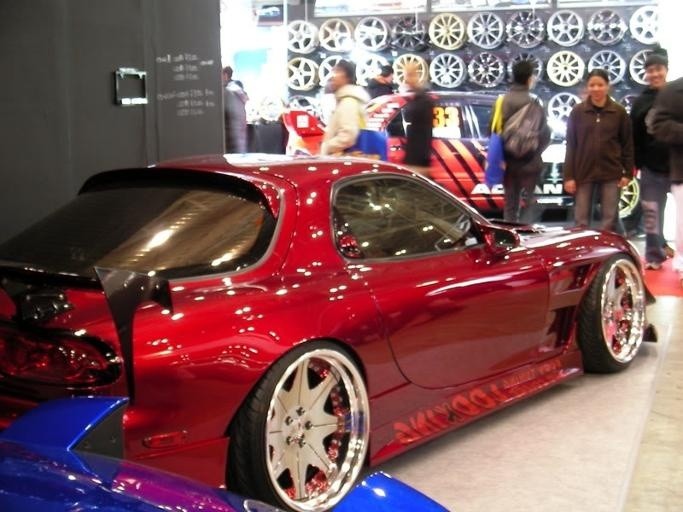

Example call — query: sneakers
[645,243,674,271]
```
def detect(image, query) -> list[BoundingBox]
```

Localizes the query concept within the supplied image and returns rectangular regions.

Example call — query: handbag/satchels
[342,126,386,160]
[501,102,542,156]
[485,132,504,185]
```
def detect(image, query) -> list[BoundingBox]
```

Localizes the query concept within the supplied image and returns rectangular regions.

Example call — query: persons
[223,66,250,153]
[367,65,395,97]
[564,69,636,233]
[321,59,370,160]
[389,76,433,177]
[648,77,683,278]
[631,49,674,270]
[491,62,550,226]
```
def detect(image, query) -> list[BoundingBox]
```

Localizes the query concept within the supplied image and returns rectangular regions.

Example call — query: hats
[644,48,668,68]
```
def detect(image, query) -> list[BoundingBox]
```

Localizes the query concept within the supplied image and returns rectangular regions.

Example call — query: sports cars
[1,153,656,512]
[282,93,639,235]
